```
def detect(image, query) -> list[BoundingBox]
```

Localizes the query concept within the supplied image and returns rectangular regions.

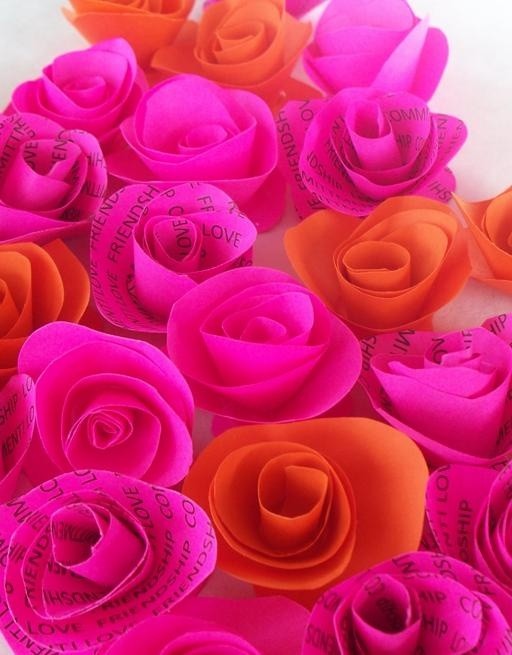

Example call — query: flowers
[0,0,512,655]
